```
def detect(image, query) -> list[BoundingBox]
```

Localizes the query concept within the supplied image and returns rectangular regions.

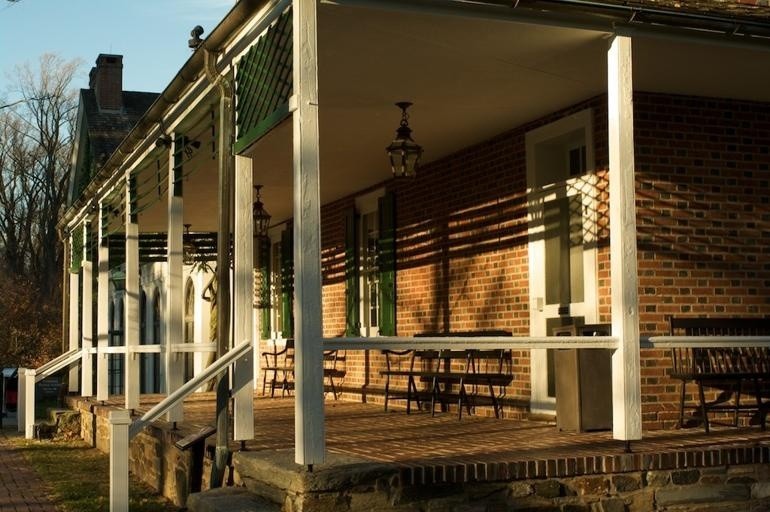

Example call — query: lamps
[110,266,126,291]
[384,100,424,184]
[182,223,196,266]
[253,183,272,237]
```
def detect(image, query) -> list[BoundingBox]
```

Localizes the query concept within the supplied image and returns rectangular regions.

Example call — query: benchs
[666,313,770,435]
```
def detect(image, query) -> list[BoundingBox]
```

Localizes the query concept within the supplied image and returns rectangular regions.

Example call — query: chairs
[379,330,513,422]
[261,333,339,401]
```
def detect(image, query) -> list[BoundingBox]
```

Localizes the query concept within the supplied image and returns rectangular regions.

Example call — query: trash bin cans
[553,323,612,433]
